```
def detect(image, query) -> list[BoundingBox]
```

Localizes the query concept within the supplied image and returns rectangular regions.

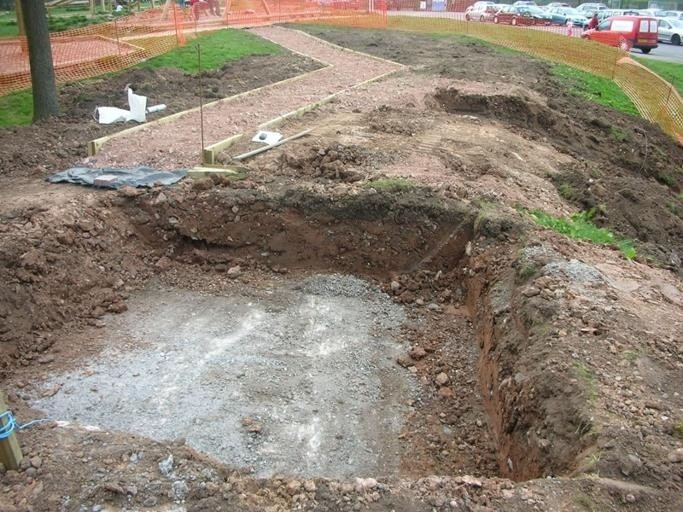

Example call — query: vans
[579,14,660,56]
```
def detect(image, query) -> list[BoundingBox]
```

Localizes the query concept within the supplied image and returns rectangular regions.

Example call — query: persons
[116,3,123,12]
[566,18,573,35]
[587,13,599,30]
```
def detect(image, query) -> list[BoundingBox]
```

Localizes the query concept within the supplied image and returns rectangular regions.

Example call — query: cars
[463,0,683,27]
[655,17,683,47]
[305,0,401,12]
